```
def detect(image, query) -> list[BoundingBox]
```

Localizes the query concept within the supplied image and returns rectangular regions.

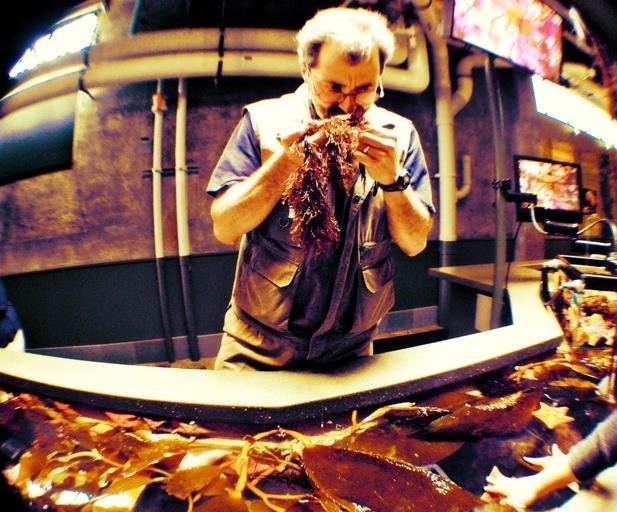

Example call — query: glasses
[304,58,385,104]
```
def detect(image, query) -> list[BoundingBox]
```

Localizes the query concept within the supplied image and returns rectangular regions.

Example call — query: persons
[481,404,617,512]
[204,5,434,375]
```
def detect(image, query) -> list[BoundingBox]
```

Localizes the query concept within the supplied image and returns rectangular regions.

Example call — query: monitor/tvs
[451,0,564,85]
[512,153,584,223]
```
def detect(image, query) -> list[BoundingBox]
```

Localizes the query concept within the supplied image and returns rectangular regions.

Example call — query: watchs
[377,167,411,192]
